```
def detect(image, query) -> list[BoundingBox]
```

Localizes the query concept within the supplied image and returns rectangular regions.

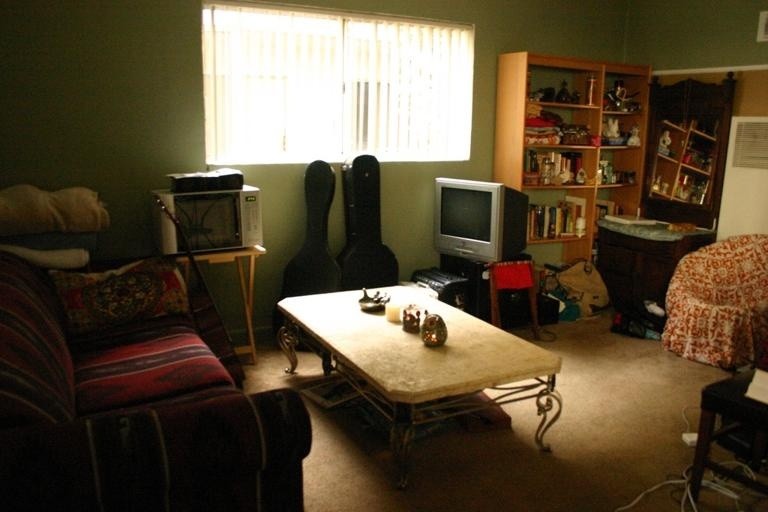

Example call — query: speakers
[411,267,469,314]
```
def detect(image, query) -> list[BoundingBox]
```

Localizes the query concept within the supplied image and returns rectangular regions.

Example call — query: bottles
[585,71,597,107]
[613,78,624,95]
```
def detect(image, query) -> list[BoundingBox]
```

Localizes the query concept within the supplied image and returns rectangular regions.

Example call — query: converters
[682,432,698,447]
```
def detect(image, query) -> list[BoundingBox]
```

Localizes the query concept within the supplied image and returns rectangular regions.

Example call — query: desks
[689,367,768,503]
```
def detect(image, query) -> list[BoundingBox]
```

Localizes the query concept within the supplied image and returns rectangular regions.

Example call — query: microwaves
[150,184,263,254]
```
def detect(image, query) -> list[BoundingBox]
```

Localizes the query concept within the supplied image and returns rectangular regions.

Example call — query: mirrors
[646,112,722,212]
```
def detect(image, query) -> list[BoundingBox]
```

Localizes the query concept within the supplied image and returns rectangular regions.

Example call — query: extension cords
[702,468,752,499]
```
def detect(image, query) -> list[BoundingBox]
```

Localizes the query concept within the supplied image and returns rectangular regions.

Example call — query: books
[593,200,624,227]
[525,148,589,240]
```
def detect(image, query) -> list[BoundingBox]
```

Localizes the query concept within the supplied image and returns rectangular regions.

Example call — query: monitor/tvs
[430,174,529,264]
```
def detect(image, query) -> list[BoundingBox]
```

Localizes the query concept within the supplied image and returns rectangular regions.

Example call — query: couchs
[1,250,312,512]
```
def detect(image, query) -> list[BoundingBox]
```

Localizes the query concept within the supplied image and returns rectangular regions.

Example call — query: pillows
[0,184,110,236]
[49,255,190,335]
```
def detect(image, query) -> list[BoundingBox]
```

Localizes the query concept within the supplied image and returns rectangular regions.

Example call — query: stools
[484,260,557,342]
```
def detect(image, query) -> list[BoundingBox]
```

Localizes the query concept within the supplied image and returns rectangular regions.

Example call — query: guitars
[154,192,246,382]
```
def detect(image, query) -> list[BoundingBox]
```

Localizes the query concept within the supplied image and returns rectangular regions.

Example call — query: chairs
[661,234,768,367]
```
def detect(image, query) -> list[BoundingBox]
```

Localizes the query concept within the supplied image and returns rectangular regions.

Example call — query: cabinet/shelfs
[590,71,737,332]
[493,51,653,264]
[648,119,717,205]
[440,253,532,329]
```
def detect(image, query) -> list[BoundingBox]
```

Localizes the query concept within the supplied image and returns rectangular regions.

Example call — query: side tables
[176,244,268,365]
